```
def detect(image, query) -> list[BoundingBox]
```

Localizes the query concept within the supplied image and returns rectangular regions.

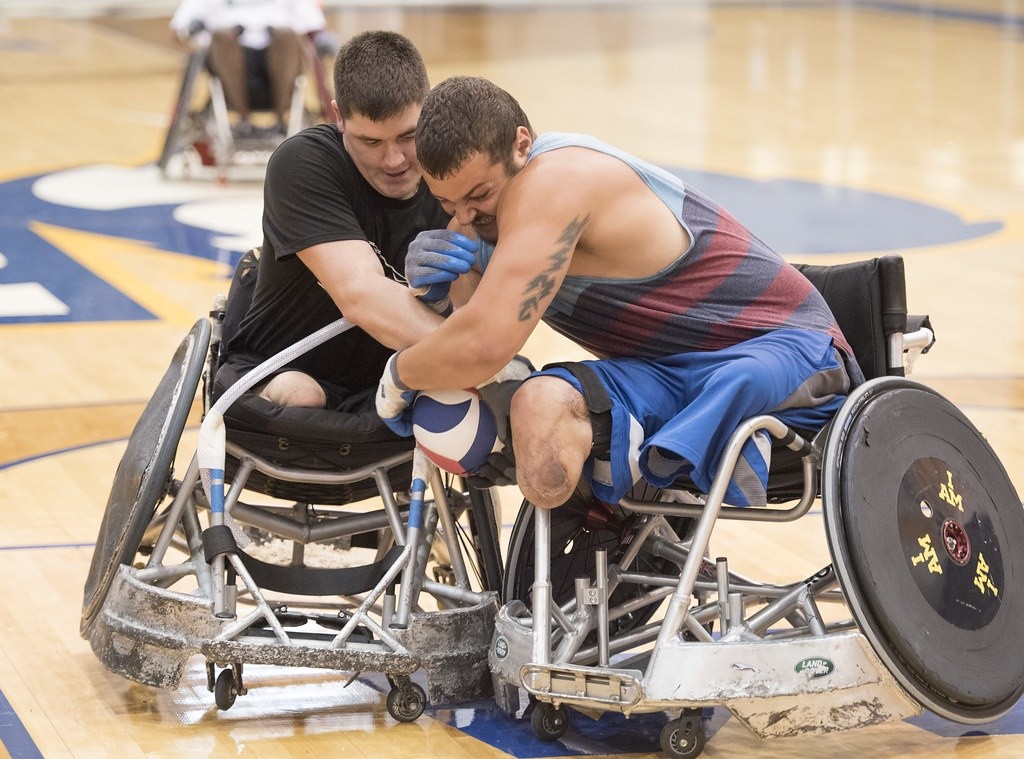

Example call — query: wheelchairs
[387,254,1024,759]
[71,247,509,725]
[158,30,347,184]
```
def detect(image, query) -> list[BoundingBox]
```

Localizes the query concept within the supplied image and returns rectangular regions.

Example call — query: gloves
[373,347,417,438]
[463,446,519,492]
[403,228,478,316]
[477,352,538,446]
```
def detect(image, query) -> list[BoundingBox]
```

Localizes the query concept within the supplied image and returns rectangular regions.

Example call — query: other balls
[408,385,506,478]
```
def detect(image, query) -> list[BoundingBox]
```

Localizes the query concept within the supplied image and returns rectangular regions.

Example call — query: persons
[373,75,863,506]
[168,1,340,137]
[205,32,466,429]
